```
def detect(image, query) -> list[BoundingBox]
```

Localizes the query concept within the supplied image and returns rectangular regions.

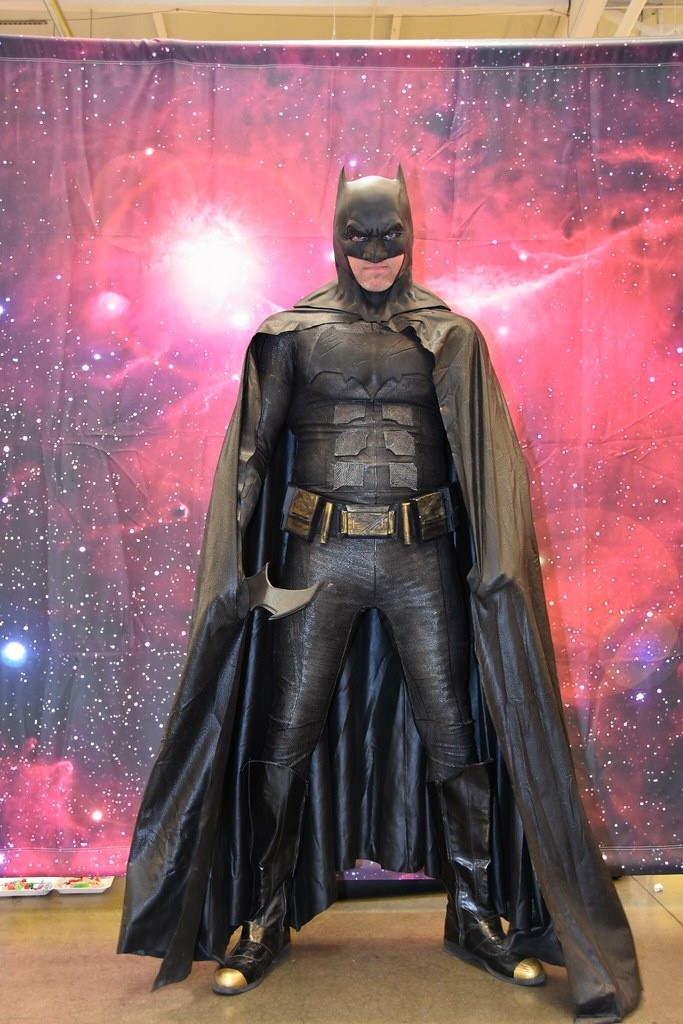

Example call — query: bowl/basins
[49,876,115,894]
[0,877,55,896]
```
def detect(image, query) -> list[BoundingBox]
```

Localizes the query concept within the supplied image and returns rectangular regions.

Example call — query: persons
[114,168,641,1012]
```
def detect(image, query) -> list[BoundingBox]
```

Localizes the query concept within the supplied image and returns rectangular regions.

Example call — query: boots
[211,754,316,995]
[427,757,547,986]
